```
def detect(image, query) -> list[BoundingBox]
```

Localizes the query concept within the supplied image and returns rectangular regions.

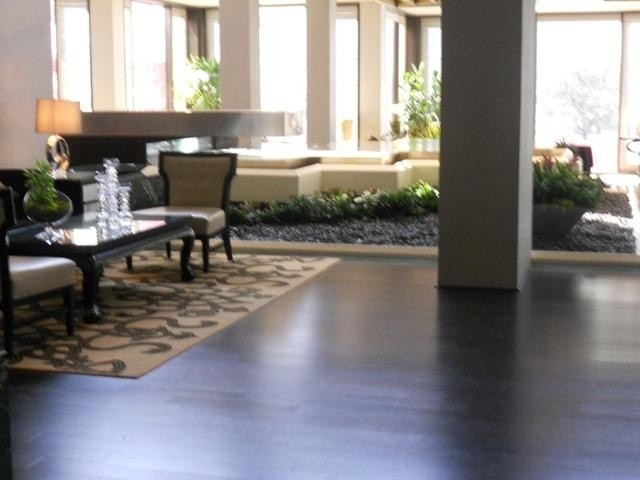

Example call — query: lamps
[35,97,82,172]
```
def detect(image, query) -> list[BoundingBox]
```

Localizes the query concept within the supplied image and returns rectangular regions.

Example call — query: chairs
[126,151,237,273]
[0,168,84,362]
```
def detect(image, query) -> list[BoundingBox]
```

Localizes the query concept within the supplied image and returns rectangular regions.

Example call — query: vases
[531,204,587,236]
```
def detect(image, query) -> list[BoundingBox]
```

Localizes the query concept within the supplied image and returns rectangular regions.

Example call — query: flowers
[533,149,611,216]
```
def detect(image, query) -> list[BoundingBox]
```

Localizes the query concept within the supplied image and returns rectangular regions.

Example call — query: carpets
[0,249,341,379]
